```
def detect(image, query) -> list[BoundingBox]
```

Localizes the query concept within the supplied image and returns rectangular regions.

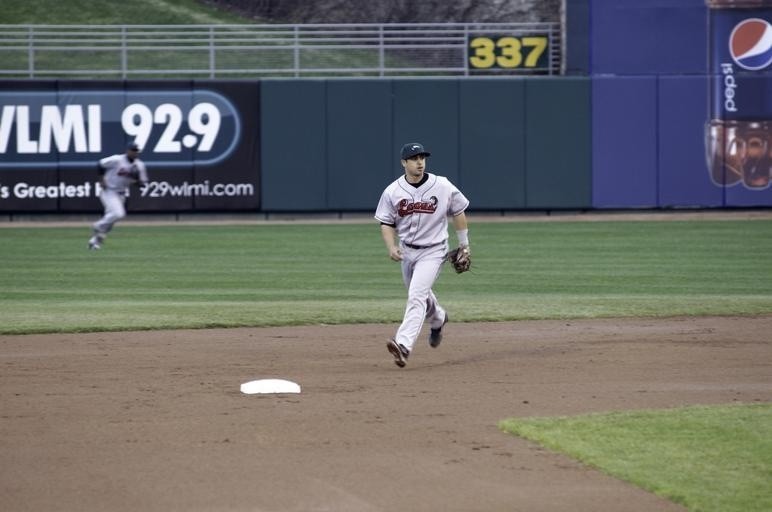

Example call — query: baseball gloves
[447,246,472,273]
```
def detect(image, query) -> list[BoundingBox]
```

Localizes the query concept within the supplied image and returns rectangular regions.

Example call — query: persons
[87,142,150,250]
[373,142,473,367]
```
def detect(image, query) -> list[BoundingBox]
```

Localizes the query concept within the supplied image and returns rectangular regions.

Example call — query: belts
[406,243,420,249]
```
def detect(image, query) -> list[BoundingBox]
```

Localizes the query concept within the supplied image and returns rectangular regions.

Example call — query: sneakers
[88,242,101,251]
[94,224,107,240]
[386,339,409,368]
[429,313,448,347]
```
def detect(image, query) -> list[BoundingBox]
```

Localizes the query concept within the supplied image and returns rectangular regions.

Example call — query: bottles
[704,1,772,191]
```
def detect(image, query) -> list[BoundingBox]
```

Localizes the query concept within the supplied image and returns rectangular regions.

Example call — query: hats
[400,143,431,160]
[127,142,139,151]
